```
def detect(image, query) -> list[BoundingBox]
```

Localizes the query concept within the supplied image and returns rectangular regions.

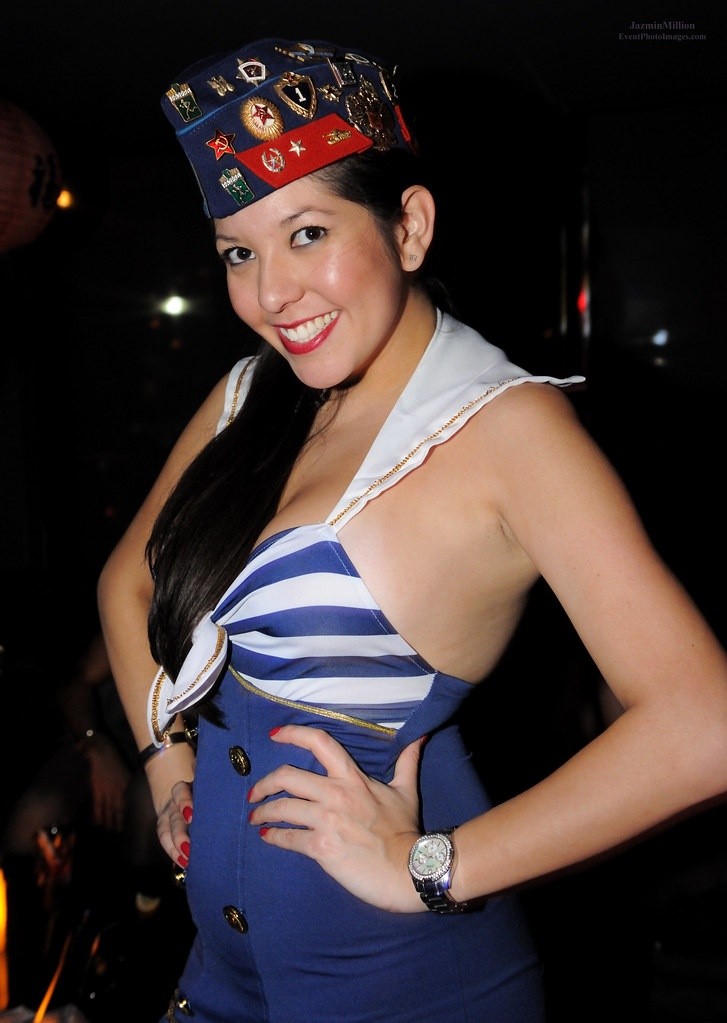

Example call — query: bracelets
[138,726,196,768]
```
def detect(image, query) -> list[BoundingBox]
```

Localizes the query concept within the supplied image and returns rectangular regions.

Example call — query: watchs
[408,826,472,915]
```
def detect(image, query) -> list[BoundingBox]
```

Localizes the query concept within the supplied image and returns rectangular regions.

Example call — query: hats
[160,38,417,220]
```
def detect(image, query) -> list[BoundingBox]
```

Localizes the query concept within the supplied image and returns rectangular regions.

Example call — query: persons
[0,634,198,1023]
[97,41,727,1022]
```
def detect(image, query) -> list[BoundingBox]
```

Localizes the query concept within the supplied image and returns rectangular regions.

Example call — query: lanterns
[0,99,60,253]
